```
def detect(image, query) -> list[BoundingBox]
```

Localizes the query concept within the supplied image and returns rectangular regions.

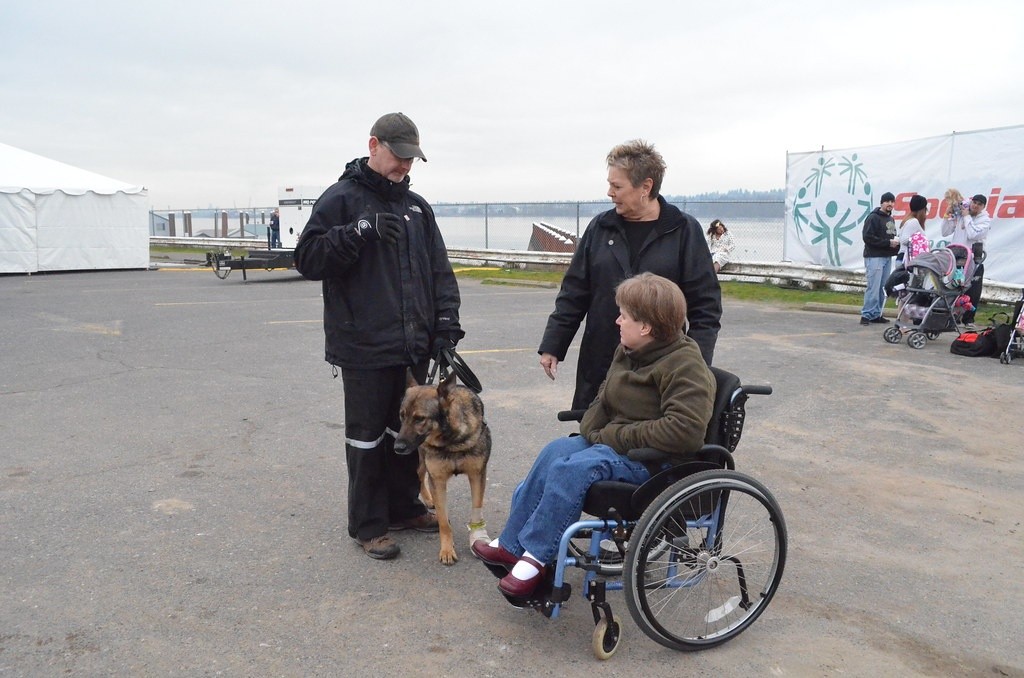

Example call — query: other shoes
[869,316,890,323]
[965,322,977,331]
[860,316,869,326]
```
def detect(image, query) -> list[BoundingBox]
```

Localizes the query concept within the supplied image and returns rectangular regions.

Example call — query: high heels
[471,539,518,573]
[497,556,550,597]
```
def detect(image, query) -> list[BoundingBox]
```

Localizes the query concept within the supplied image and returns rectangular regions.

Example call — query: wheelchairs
[480,363,789,663]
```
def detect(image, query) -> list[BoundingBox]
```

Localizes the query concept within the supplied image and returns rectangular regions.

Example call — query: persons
[707,220,736,273]
[294,115,460,558]
[472,277,717,598]
[270,208,280,248]
[941,195,991,327]
[861,192,898,325]
[538,141,723,556]
[896,195,928,334]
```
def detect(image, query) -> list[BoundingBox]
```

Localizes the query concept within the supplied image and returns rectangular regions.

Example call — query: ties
[909,195,928,211]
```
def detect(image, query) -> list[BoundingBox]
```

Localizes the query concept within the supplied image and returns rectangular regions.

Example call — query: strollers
[999,300,1024,366]
[881,241,988,350]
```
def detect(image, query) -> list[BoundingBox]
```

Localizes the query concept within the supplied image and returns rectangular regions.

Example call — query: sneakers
[354,532,400,560]
[385,509,451,532]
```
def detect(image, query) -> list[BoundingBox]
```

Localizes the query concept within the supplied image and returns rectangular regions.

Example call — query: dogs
[392,364,493,566]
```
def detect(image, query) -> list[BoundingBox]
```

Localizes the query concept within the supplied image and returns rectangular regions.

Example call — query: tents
[0,142,150,273]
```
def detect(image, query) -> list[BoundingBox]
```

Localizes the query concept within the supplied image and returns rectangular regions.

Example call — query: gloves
[351,212,402,247]
[432,336,455,367]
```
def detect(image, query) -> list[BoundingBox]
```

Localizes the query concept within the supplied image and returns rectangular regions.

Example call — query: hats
[880,192,896,204]
[370,112,427,162]
[972,194,986,205]
